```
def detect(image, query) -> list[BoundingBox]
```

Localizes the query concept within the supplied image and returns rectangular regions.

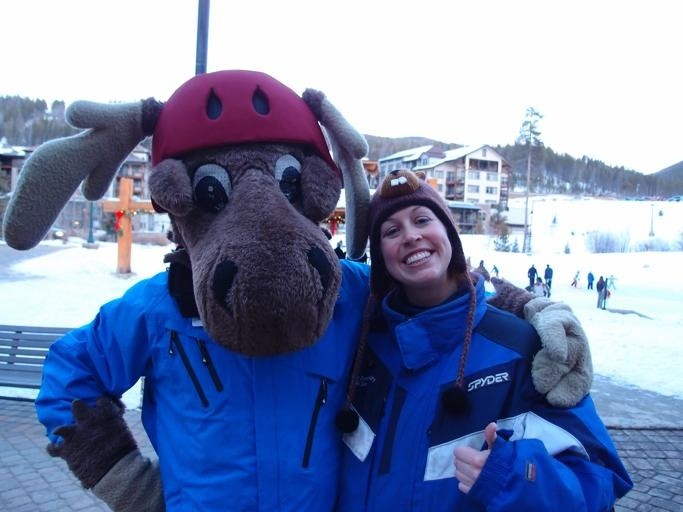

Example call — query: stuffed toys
[4,68,594,512]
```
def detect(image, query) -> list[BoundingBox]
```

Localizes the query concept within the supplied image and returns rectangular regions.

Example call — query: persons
[462,254,619,311]
[342,162,634,511]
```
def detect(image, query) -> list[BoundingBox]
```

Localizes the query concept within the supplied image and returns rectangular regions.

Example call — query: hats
[365,169,468,297]
